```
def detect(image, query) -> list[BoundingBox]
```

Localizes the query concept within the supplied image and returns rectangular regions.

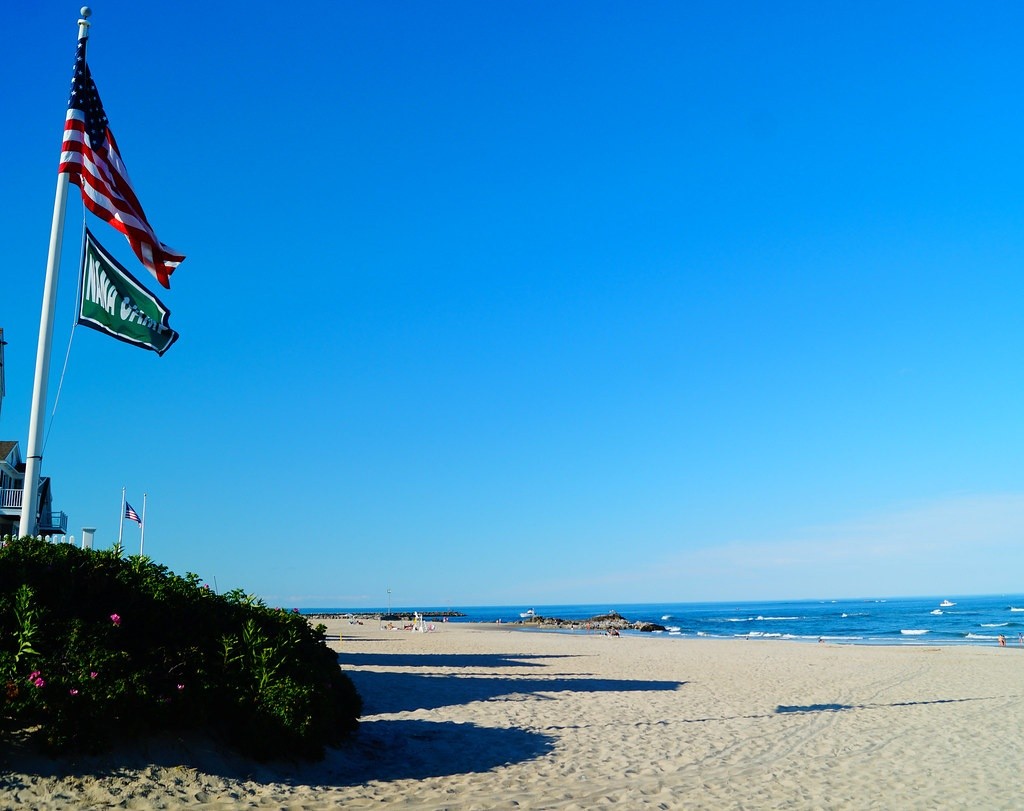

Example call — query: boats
[900,630,930,636]
[519,607,536,618]
[1010,606,1024,612]
[965,632,1015,639]
[939,600,957,607]
[980,622,1008,629]
[930,609,944,615]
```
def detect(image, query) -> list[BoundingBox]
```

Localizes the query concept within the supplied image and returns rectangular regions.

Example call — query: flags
[124,501,142,529]
[77,226,179,357]
[58,36,186,290]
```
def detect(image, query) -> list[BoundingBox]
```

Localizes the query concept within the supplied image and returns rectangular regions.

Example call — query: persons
[998,634,1006,646]
[1019,632,1023,643]
